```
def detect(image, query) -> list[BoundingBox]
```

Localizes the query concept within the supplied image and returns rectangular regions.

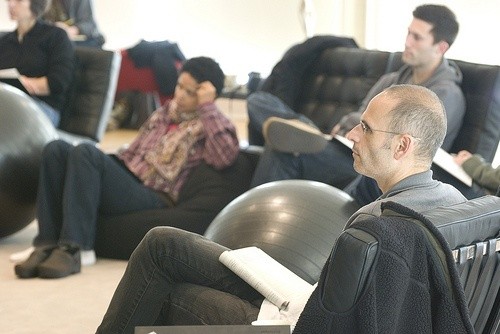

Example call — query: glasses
[360,121,421,141]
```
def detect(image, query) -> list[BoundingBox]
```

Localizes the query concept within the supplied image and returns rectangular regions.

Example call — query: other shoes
[263,116,328,153]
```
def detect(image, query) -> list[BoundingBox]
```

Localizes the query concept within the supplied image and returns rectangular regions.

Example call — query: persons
[95,84,467,333]
[13,57,240,278]
[246,5,466,185]
[342,140,500,205]
[30,0,105,47]
[0,0,75,128]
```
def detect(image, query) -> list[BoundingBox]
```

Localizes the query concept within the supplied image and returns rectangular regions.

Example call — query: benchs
[230,46,500,200]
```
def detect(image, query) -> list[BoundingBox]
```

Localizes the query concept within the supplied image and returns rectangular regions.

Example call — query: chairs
[55,47,123,148]
[317,192,500,334]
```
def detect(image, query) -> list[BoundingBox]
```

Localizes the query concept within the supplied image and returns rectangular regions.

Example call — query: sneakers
[15,250,49,279]
[37,247,80,279]
